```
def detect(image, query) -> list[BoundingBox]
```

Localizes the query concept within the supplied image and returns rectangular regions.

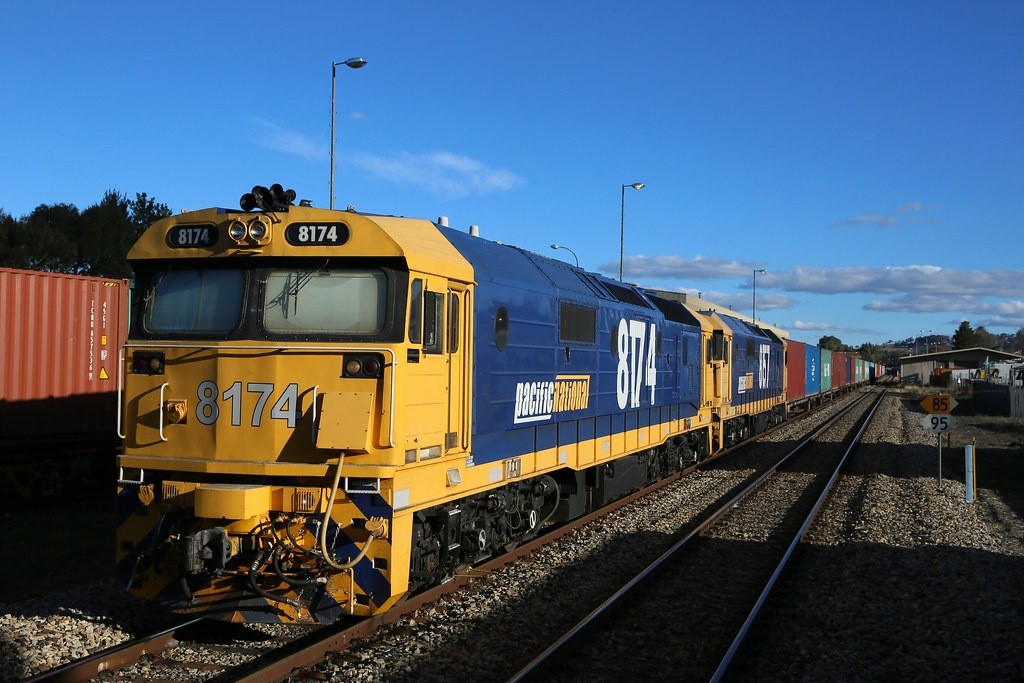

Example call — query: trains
[113,184,886,627]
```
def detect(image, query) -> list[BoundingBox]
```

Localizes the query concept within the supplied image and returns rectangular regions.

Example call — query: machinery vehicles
[975,362,999,380]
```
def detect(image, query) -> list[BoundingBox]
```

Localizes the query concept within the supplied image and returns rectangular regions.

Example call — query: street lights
[330,57,368,209]
[934,342,941,353]
[753,269,768,320]
[620,182,646,282]
[919,329,933,354]
